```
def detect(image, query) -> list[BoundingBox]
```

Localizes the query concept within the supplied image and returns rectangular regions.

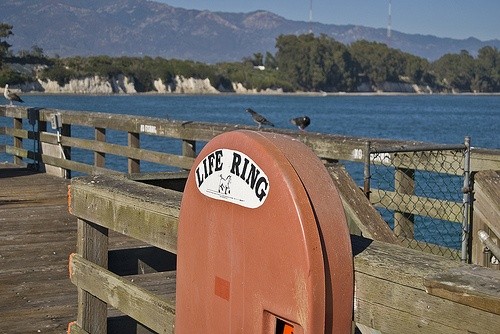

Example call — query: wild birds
[4,84,25,106]
[244,108,276,130]
[288,115,311,133]
